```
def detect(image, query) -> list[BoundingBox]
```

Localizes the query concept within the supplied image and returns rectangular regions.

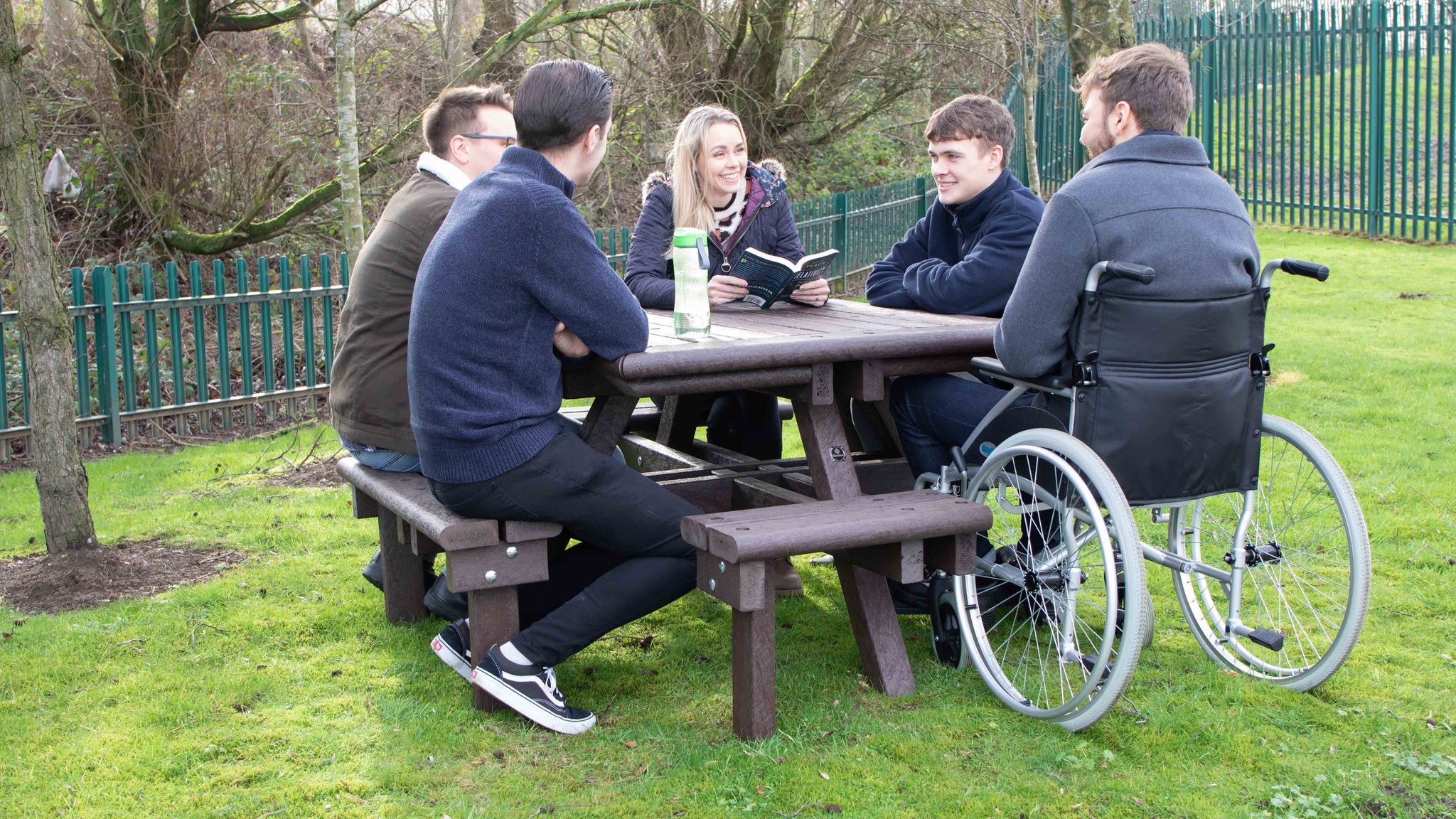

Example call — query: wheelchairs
[913,259,1369,732]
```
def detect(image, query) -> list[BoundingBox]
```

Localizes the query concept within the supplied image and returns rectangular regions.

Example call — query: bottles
[673,227,711,338]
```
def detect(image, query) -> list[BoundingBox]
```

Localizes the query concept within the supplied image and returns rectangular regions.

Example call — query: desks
[584,288,1002,702]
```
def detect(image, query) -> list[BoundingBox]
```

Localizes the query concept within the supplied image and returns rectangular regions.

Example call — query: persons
[406,59,706,734]
[328,82,520,622]
[624,103,832,595]
[839,93,1044,465]
[890,43,1260,630]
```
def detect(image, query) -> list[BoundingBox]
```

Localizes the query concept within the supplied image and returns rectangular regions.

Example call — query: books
[730,248,839,313]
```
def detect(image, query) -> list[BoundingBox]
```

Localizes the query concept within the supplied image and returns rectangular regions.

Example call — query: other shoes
[771,554,803,596]
[420,570,469,620]
[892,572,995,631]
[997,543,1060,624]
[360,544,433,599]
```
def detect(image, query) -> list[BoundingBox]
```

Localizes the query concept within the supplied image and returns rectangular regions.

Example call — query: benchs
[559,399,794,460]
[331,450,564,716]
[674,487,996,747]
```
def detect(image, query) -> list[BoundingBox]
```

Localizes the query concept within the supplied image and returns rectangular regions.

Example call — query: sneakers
[471,644,597,737]
[429,618,473,684]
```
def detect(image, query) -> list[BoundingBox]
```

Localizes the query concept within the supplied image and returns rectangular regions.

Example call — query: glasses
[447,135,516,151]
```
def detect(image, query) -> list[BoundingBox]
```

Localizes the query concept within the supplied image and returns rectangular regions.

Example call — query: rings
[814,295,819,303]
